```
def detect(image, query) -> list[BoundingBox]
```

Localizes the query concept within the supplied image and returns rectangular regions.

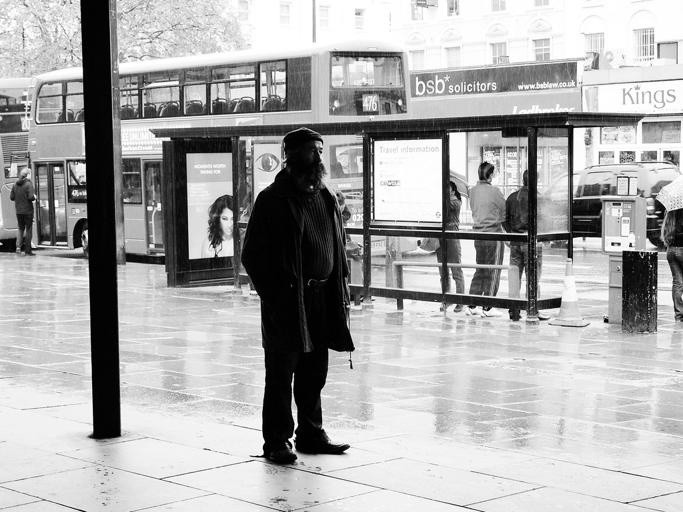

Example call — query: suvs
[504,161,683,251]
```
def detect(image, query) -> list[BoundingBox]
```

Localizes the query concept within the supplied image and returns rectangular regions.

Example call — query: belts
[303,275,337,291]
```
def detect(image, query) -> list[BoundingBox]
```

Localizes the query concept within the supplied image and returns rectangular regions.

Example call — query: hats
[283,127,325,155]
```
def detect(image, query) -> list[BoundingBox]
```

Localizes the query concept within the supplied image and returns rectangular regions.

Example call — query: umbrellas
[656,173,683,212]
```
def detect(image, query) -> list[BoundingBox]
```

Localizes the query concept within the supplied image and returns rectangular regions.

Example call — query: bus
[1,77,68,243]
[8,47,420,266]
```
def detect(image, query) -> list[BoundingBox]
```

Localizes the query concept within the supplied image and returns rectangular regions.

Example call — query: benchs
[391,259,520,310]
[346,255,363,288]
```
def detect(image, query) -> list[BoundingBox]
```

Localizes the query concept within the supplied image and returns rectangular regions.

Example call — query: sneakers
[262,437,298,466]
[295,430,351,455]
[16,247,37,257]
[440,301,551,326]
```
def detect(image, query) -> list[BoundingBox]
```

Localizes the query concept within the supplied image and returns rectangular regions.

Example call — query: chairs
[51,93,286,128]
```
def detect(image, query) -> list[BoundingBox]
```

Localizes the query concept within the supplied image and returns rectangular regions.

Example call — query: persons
[434,180,465,312]
[9,167,37,256]
[664,207,683,324]
[500,169,551,321]
[240,128,355,465]
[334,190,351,286]
[467,162,506,317]
[204,194,235,259]
